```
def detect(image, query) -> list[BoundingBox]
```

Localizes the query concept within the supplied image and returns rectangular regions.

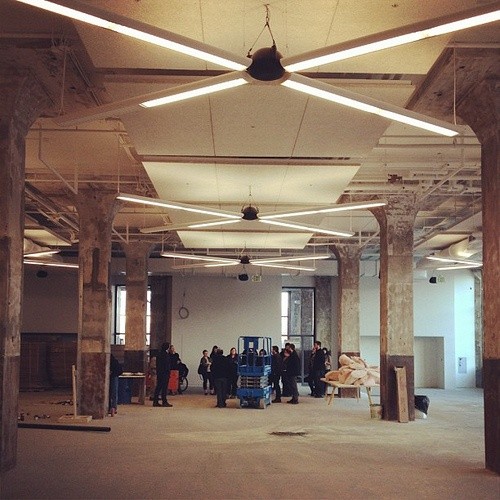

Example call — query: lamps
[18,0,500,137]
[24,249,79,268]
[159,247,329,272]
[426,256,484,270]
[116,186,388,239]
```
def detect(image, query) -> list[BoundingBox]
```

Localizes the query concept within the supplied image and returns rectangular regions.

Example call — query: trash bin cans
[414,395,430,416]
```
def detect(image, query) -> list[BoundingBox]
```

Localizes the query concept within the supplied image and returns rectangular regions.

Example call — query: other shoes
[162,402,172,407]
[153,402,162,406]
[286,398,298,404]
[210,392,216,395]
[272,398,281,403]
[315,394,323,398]
[204,392,208,395]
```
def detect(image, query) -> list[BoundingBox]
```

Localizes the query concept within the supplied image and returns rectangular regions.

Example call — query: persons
[153,341,182,407]
[108,353,123,415]
[307,340,332,399]
[198,343,301,408]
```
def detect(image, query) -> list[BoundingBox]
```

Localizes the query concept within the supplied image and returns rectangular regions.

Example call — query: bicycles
[178,375,188,392]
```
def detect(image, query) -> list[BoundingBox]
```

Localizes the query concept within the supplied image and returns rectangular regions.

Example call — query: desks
[119,375,146,404]
[320,378,360,405]
